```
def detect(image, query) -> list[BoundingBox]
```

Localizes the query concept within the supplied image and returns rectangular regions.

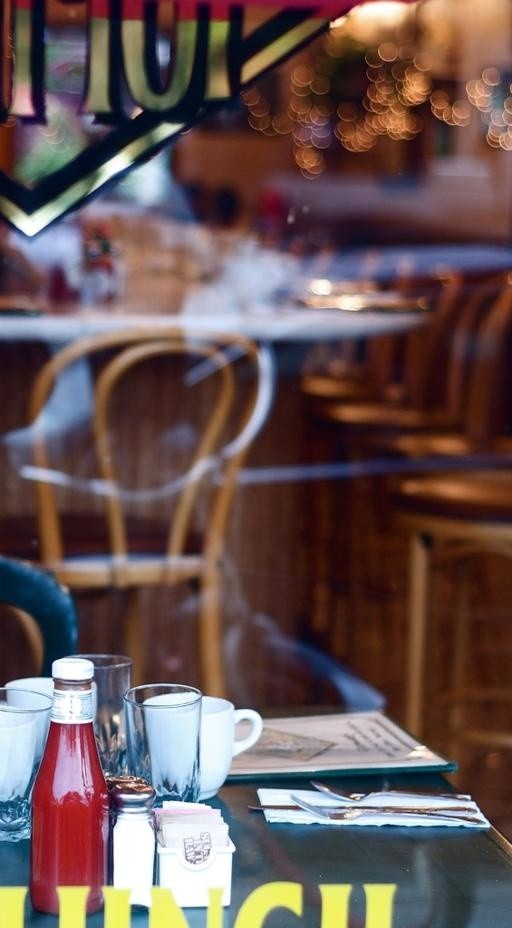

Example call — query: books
[216,706,462,787]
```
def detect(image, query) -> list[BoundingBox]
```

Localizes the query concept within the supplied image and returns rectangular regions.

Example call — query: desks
[4,712,512,927]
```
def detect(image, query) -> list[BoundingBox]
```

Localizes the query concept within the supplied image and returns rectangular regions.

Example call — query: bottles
[106,773,156,842]
[27,658,155,914]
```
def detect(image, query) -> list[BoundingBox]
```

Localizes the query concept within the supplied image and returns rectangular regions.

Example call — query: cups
[121,682,202,810]
[87,659,148,780]
[142,694,263,801]
[2,688,55,841]
[3,677,97,749]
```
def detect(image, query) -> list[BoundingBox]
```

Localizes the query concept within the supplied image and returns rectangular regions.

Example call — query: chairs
[262,231,512,842]
[0,327,259,699]
